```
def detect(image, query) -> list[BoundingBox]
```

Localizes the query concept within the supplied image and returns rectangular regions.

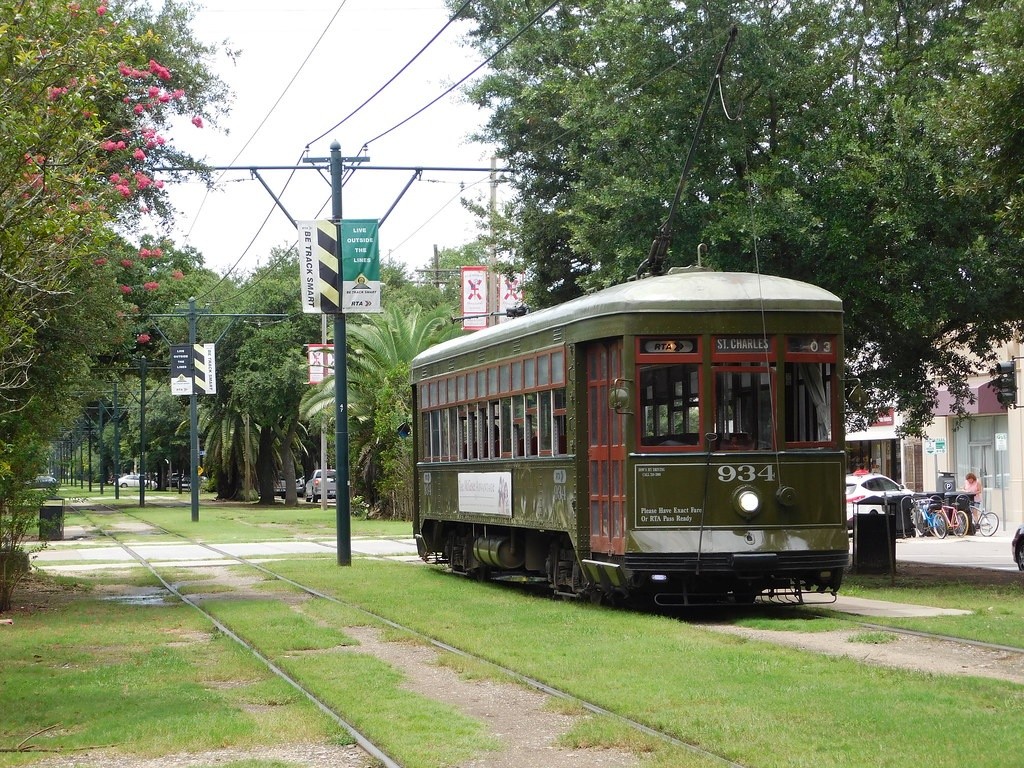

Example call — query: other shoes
[975,524,980,528]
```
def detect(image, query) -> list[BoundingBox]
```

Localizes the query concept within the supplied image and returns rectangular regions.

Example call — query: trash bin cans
[944,491,976,535]
[37,494,65,542]
[852,496,898,574]
[912,492,946,537]
[882,494,917,539]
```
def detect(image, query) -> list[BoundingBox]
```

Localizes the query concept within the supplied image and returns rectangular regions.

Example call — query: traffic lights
[995,362,1016,406]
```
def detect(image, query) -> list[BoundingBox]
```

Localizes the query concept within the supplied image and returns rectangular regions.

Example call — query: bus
[407,244,849,615]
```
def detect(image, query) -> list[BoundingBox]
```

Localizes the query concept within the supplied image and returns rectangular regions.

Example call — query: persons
[855,463,868,477]
[962,473,982,524]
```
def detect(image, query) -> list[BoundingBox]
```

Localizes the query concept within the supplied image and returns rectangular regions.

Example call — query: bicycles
[911,494,1000,539]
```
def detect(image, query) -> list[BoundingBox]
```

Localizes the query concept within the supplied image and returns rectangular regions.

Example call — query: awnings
[923,378,1008,416]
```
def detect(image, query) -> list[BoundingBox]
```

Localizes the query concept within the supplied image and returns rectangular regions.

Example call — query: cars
[22,475,59,488]
[166,474,208,489]
[844,469,920,528]
[115,474,157,489]
[1011,525,1024,570]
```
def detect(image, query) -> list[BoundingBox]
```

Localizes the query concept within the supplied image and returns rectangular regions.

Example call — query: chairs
[459,436,566,460]
[640,433,753,452]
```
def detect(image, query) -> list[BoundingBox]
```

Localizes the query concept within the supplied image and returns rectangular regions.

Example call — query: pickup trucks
[306,469,338,504]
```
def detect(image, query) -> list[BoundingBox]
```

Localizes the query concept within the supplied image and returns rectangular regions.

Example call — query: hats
[966,473,976,480]
